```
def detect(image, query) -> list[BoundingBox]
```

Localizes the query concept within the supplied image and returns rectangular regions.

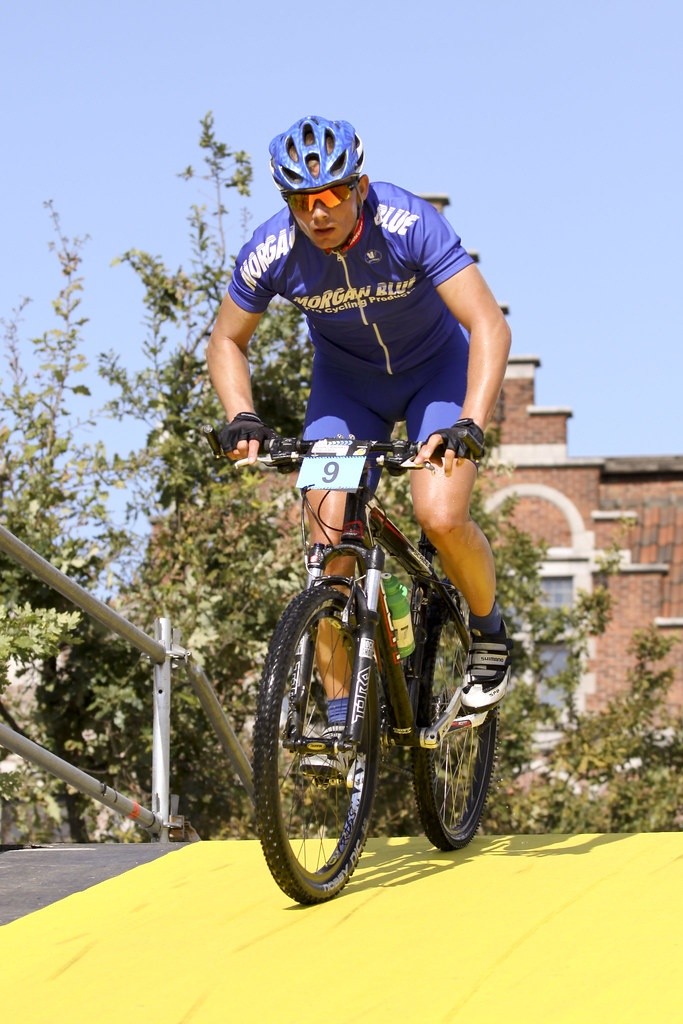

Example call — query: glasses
[280,173,359,213]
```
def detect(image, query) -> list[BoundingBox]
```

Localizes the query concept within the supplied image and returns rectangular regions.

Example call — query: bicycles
[232,430,498,905]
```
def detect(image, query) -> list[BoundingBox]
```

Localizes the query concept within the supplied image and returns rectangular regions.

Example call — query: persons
[207,115,515,779]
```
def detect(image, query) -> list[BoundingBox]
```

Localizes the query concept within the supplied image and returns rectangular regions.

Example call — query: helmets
[269,115,364,194]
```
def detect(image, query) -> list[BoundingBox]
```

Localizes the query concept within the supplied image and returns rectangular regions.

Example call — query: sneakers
[299,726,355,781]
[460,618,515,714]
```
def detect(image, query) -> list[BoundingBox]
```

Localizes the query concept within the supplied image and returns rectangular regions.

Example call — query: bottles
[381,572,416,658]
